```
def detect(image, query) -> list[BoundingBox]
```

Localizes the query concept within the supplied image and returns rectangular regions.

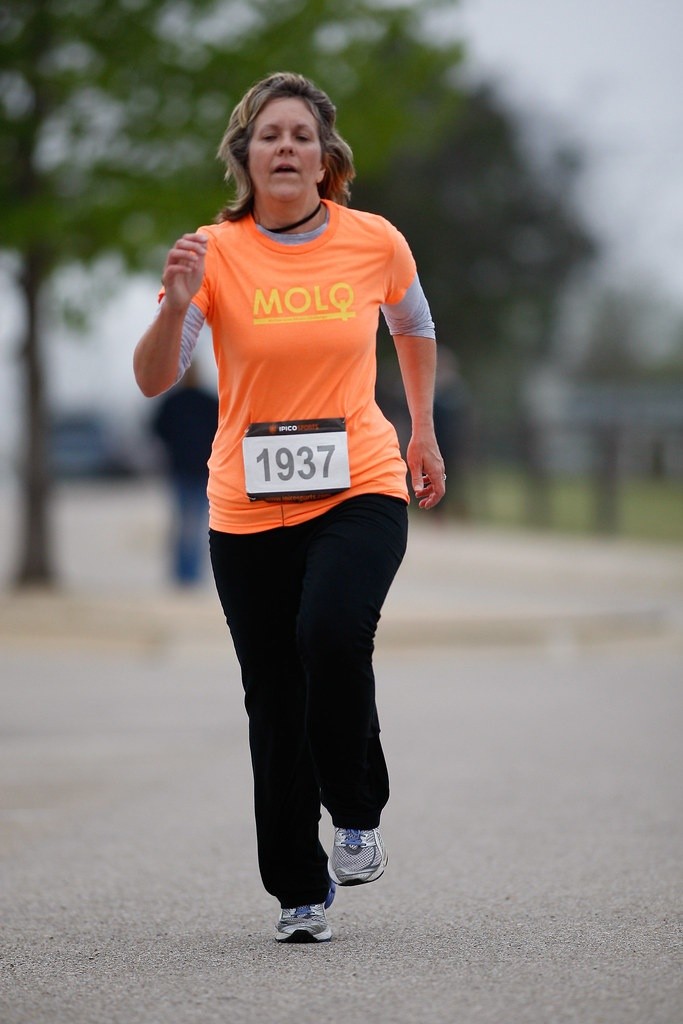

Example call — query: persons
[128,73,446,943]
[150,357,218,583]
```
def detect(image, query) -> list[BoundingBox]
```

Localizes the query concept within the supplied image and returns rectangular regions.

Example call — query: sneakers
[274,882,336,943]
[327,827,388,886]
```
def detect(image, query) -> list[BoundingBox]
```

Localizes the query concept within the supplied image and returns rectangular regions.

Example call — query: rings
[444,474,446,480]
